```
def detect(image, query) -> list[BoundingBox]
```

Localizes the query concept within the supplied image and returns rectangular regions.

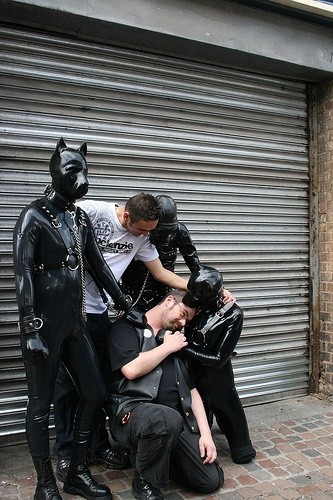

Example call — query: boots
[63,467,112,500]
[34,477,63,500]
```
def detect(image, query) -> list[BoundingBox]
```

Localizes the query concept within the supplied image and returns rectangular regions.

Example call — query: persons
[105,287,226,500]
[53,191,237,473]
[155,264,257,465]
[12,136,138,500]
[118,194,202,314]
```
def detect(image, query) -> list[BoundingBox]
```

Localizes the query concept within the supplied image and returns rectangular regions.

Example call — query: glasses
[170,293,190,327]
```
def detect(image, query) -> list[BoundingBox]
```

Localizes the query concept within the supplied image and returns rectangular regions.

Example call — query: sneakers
[91,448,128,469]
[56,456,71,483]
[131,470,165,500]
